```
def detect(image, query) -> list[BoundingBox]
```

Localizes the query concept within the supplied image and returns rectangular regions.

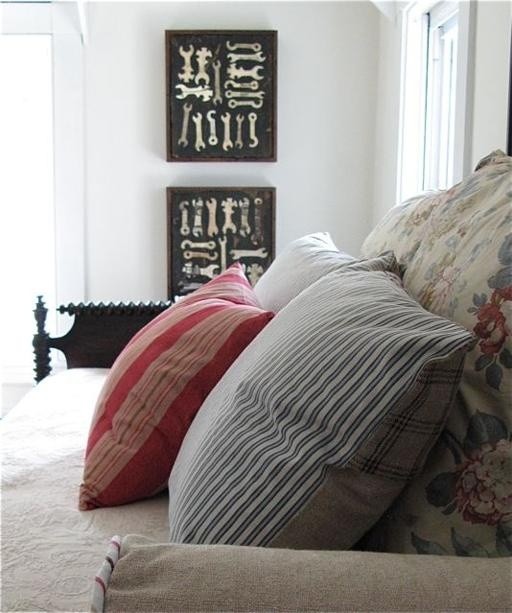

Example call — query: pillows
[83,263,275,516]
[357,154,510,558]
[250,229,359,311]
[164,255,473,546]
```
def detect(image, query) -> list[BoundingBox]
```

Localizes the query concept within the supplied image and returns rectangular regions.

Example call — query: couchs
[0,294,511,611]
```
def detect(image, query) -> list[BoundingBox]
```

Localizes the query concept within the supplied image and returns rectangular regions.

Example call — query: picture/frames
[166,188,276,301]
[165,29,277,162]
